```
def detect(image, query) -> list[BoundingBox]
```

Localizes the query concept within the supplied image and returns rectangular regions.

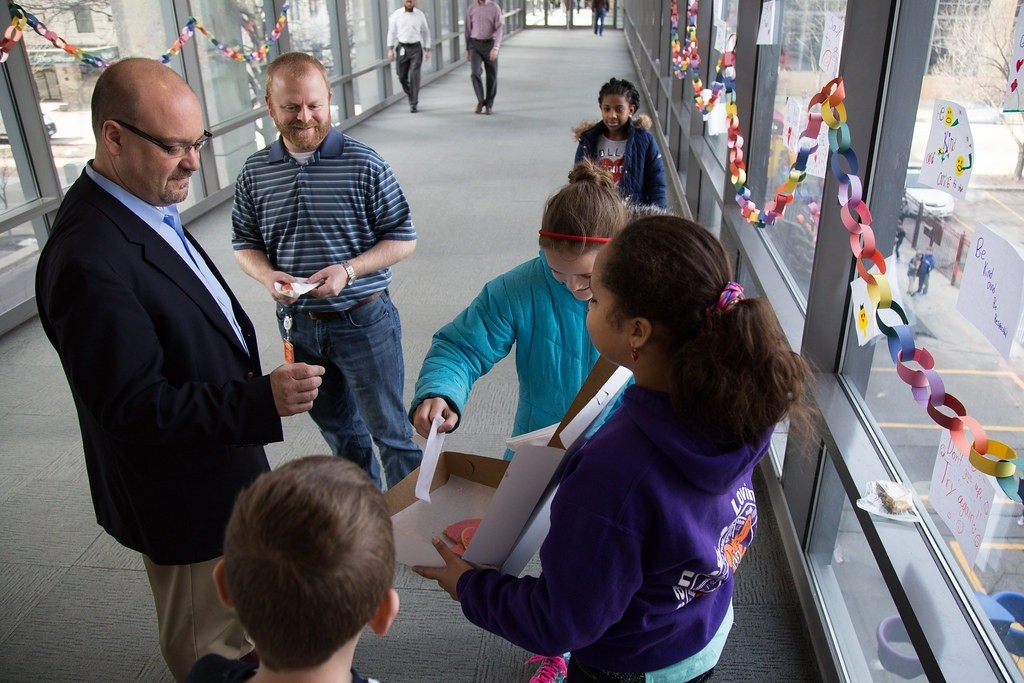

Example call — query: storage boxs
[378,352,633,577]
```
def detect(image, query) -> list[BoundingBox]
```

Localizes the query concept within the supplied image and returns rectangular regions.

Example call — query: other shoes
[410,105,416,112]
[475,101,485,113]
[485,105,493,114]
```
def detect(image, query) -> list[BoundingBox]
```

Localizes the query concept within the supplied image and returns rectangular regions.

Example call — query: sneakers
[524,653,571,683]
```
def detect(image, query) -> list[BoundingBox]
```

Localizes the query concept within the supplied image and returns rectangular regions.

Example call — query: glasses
[111,118,213,158]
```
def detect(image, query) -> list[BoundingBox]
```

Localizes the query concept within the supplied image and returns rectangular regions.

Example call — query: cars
[926,36,979,75]
[0,112,58,143]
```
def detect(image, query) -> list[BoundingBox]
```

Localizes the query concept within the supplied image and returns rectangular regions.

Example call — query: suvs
[902,161,955,224]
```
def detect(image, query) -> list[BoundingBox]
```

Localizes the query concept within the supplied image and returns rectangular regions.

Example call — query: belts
[399,42,420,47]
[470,37,493,42]
[294,290,384,321]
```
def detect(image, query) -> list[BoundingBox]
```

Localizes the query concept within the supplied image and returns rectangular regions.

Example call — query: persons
[408,159,636,459]
[185,454,398,683]
[230,52,423,491]
[36,57,325,683]
[387,0,430,113]
[592,0,610,37]
[907,248,934,295]
[465,0,503,114]
[574,78,668,209]
[411,216,819,683]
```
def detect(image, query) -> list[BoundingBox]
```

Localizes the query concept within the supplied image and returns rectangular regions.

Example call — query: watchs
[343,262,357,285]
